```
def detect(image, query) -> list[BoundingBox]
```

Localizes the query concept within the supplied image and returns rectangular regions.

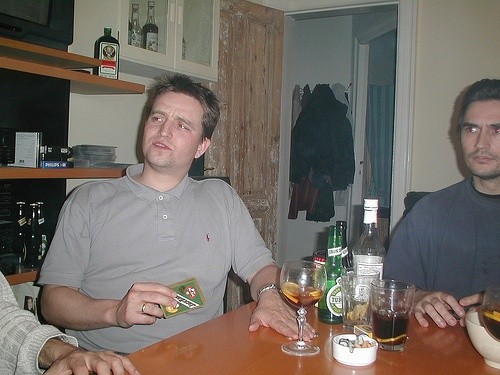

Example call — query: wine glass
[279,260,327,357]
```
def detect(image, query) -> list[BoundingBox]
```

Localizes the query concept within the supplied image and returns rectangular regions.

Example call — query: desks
[120,301,500,375]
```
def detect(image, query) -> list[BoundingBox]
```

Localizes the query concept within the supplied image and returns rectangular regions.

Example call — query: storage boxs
[354,324,373,338]
[159,277,206,318]
[1,129,75,168]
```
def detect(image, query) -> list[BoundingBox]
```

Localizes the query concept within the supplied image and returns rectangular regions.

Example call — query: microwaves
[0,0,74,52]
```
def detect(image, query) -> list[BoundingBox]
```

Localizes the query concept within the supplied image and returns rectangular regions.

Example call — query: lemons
[483,309,500,322]
[346,302,368,321]
[281,282,321,304]
[375,334,406,342]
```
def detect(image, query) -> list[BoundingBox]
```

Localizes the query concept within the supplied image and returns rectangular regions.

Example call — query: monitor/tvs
[0,0,75,52]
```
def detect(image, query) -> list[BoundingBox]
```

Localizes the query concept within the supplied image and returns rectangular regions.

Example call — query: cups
[369,279,415,352]
[339,267,383,329]
[480,286,500,341]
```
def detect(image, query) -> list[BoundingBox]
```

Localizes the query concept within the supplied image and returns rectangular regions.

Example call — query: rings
[142,303,146,314]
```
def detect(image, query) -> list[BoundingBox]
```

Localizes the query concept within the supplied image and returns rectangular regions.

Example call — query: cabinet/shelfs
[0,37,145,286]
[108,0,220,84]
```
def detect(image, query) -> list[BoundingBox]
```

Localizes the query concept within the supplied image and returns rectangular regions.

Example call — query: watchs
[258,282,282,295]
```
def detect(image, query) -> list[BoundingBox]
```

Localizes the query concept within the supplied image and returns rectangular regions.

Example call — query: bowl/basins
[465,312,500,369]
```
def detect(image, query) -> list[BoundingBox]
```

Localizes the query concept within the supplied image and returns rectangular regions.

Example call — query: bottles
[92,27,120,81]
[126,4,145,49]
[12,202,49,266]
[335,222,353,272]
[142,1,159,52]
[316,225,348,323]
[352,199,387,305]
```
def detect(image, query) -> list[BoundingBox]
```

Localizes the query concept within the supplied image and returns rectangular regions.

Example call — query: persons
[0,271,141,375]
[37,71,315,357]
[383,77,500,328]
